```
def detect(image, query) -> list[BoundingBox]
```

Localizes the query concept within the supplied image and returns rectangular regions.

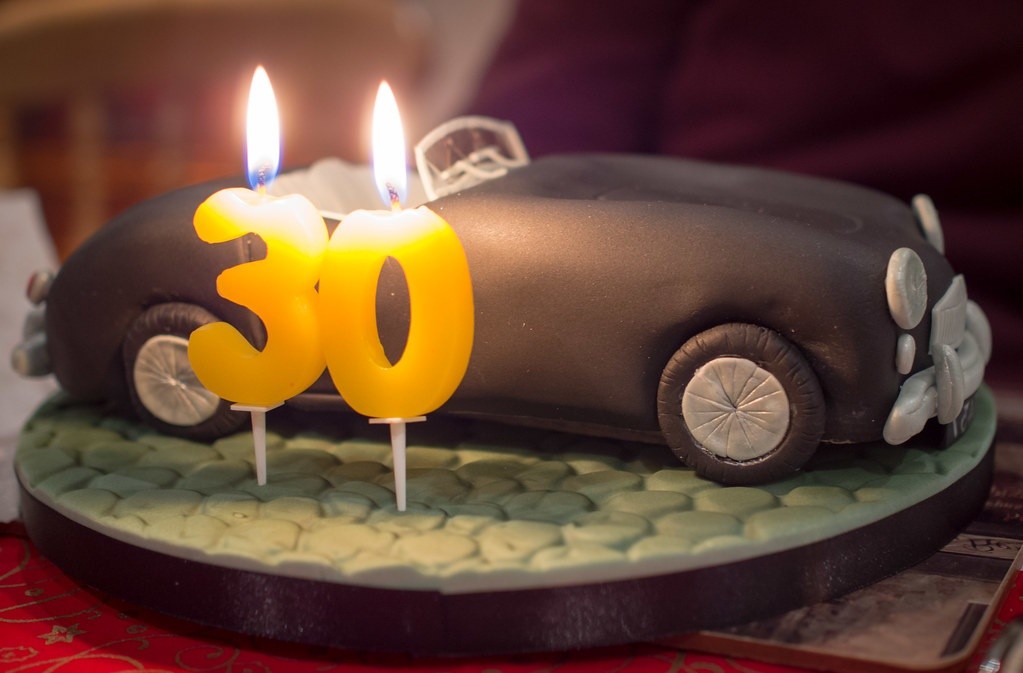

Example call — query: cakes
[7,115,1000,591]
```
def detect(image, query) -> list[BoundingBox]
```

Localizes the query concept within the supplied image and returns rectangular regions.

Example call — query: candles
[188,65,327,488]
[318,79,475,512]
